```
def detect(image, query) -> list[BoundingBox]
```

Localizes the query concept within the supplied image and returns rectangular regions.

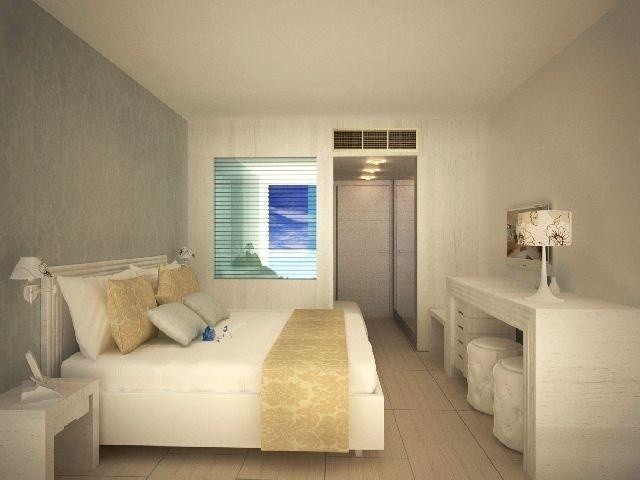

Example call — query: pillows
[106,276,159,355]
[184,292,230,326]
[155,265,199,304]
[56,270,136,360]
[129,260,181,292]
[145,302,207,346]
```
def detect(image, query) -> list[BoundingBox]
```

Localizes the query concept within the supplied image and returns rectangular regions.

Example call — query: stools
[493,357,524,454]
[466,336,522,414]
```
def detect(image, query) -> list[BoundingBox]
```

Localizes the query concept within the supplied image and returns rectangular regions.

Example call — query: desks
[0,378,100,480]
[429,309,445,367]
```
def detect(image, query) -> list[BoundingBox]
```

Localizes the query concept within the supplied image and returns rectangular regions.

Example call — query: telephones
[20,351,62,403]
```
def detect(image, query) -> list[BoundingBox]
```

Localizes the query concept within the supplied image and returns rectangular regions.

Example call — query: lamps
[516,211,572,304]
[245,243,254,252]
[9,256,57,302]
[178,247,195,266]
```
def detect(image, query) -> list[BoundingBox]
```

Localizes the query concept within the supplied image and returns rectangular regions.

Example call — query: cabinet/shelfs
[443,274,640,480]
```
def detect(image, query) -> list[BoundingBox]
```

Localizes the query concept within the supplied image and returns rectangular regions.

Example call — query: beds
[41,255,384,456]
